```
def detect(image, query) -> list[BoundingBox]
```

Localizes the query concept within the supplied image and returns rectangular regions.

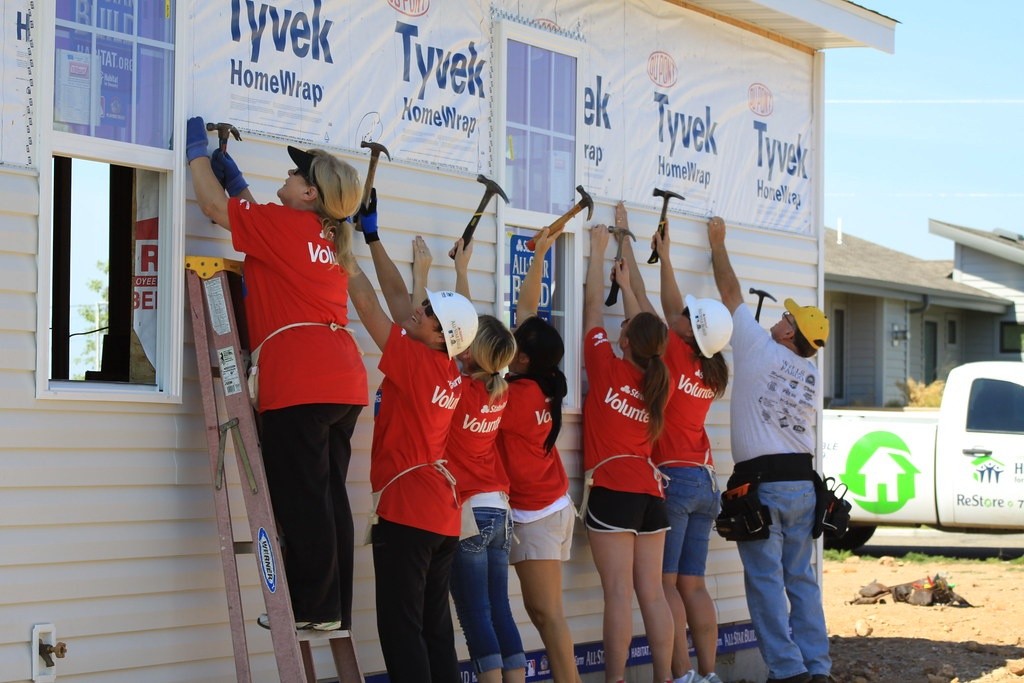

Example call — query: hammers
[206,121,243,225]
[447,173,510,262]
[748,287,778,323]
[605,225,637,308]
[646,187,686,265]
[525,184,594,252]
[355,140,391,232]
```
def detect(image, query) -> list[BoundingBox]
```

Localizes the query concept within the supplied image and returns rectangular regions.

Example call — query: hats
[287,146,361,224]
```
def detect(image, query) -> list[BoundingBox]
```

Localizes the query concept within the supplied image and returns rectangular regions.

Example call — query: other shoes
[812,674,834,683]
[767,672,812,683]
[254,614,343,632]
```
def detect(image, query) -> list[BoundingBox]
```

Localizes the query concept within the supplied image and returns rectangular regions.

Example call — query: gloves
[359,188,380,244]
[187,116,208,163]
[210,148,250,198]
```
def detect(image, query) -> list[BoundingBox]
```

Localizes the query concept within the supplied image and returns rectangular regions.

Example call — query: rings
[714,223,718,225]
[618,221,620,223]
[420,250,425,253]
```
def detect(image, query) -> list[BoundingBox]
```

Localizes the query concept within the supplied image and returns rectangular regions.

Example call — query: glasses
[293,167,310,182]
[422,299,442,333]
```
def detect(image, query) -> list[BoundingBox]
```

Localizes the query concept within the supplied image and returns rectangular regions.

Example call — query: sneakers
[672,670,710,683]
[705,672,723,683]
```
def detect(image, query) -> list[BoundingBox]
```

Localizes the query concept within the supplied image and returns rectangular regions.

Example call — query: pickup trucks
[815,358,1024,547]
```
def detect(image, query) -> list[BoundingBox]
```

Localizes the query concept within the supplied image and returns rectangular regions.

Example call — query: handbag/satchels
[715,478,772,543]
[811,470,852,540]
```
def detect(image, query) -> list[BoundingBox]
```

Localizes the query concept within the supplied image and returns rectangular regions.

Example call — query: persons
[170,116,368,631]
[338,189,478,683]
[707,215,831,683]
[614,202,737,683]
[583,224,675,683]
[410,235,528,683]
[498,227,586,683]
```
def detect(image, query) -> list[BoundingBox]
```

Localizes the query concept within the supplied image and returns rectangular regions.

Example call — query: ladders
[183,254,366,683]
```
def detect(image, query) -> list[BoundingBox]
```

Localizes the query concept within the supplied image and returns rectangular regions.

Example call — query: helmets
[784,298,829,350]
[685,294,733,359]
[425,289,479,359]
